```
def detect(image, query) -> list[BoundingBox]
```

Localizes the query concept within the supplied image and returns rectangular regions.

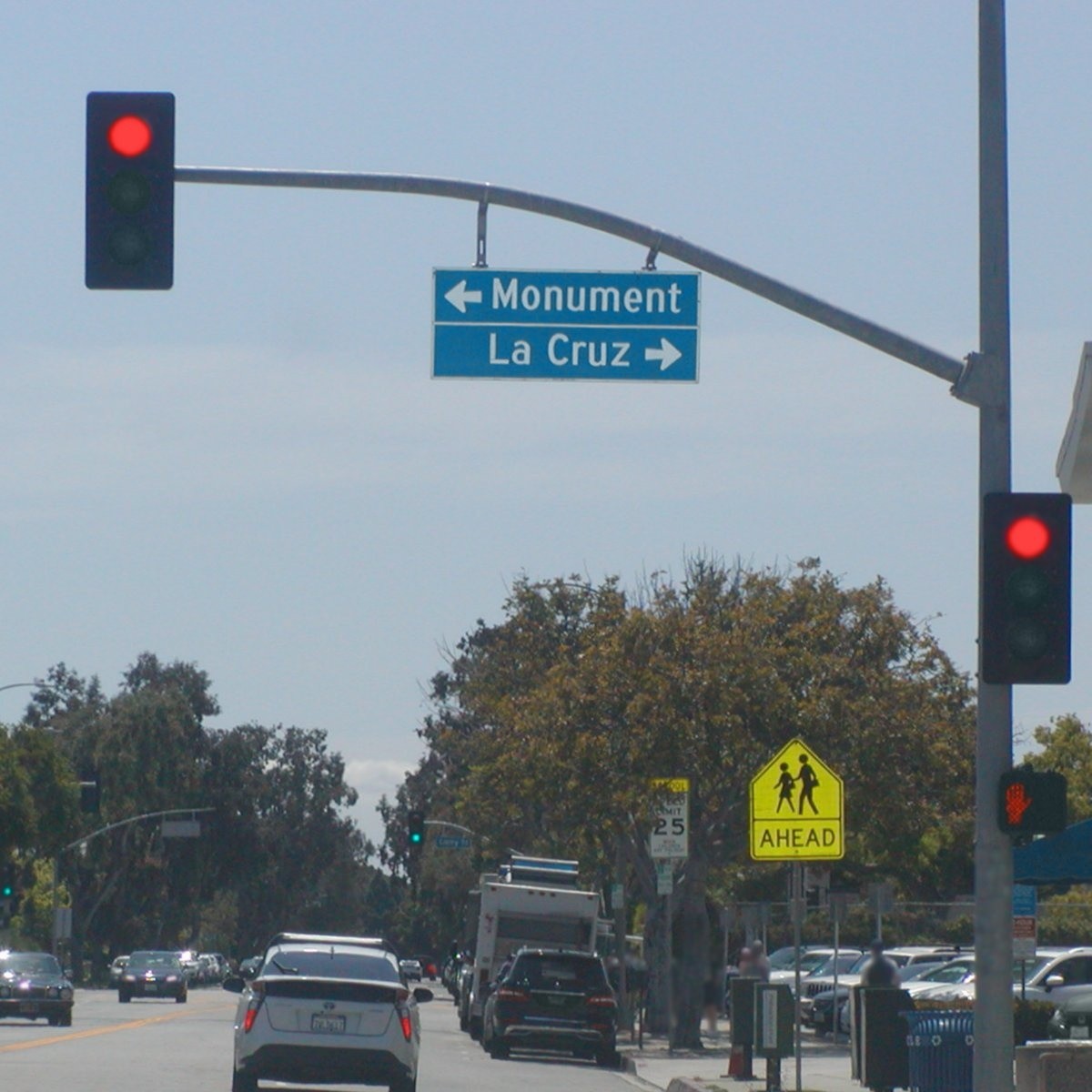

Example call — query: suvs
[480,942,622,1068]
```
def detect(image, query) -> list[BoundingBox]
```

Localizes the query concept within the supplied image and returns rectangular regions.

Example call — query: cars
[398,959,424,982]
[236,931,403,990]
[0,951,75,1027]
[116,950,195,1003]
[722,943,978,1052]
[911,945,1092,1041]
[176,950,231,989]
[438,951,475,1033]
[107,955,131,990]
[223,942,434,1092]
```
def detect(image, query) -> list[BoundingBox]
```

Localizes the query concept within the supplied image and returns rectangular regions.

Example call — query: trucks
[458,856,604,1046]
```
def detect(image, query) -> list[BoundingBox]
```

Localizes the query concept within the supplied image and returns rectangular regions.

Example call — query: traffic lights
[84,92,176,289]
[996,770,1068,833]
[979,491,1074,685]
[1,873,13,898]
[408,809,425,847]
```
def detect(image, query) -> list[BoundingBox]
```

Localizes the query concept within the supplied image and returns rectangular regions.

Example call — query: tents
[1014,819,1092,1000]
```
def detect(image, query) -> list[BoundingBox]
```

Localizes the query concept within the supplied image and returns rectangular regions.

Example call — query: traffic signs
[433,267,699,383]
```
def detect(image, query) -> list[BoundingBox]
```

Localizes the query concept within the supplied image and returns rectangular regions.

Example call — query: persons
[860,939,902,989]
[740,939,771,984]
[701,912,725,1040]
